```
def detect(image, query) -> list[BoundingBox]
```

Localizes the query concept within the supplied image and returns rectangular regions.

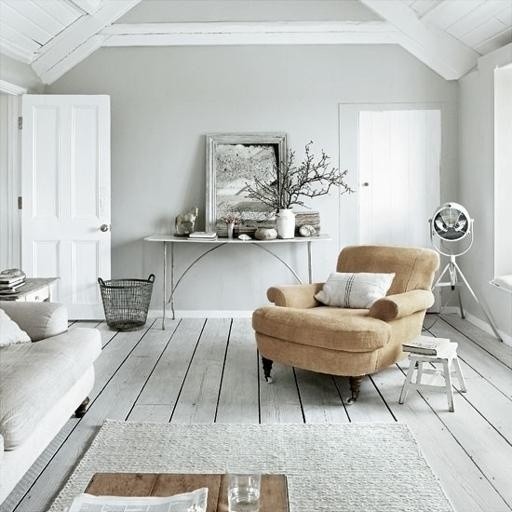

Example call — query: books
[0,274,26,291]
[187,231,218,241]
[400,336,450,356]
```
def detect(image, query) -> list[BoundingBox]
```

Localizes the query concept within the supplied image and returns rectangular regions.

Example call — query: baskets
[97,274,155,331]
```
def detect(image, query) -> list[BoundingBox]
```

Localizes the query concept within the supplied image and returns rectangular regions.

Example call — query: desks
[84,472,290,512]
[0,277,63,301]
[143,233,333,330]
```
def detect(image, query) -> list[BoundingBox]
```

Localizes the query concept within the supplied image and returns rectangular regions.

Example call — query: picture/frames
[205,132,288,237]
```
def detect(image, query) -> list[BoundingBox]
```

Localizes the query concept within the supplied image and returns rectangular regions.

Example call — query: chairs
[251,245,441,404]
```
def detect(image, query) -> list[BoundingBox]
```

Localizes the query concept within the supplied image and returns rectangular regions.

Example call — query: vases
[276,208,297,238]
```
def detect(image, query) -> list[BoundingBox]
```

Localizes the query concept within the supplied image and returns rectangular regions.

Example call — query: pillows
[0,308,31,347]
[313,271,396,308]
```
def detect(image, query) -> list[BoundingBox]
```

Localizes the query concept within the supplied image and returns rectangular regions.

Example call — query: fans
[427,201,504,343]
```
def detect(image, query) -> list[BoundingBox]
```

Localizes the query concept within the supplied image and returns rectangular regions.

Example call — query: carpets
[47,419,456,512]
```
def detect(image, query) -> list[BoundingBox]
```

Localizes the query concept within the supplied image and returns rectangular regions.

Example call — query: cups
[226,463,261,512]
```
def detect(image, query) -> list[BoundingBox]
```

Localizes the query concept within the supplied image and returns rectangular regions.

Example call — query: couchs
[0,301,102,505]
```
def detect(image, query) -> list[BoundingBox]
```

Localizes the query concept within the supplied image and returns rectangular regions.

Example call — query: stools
[399,342,467,412]
